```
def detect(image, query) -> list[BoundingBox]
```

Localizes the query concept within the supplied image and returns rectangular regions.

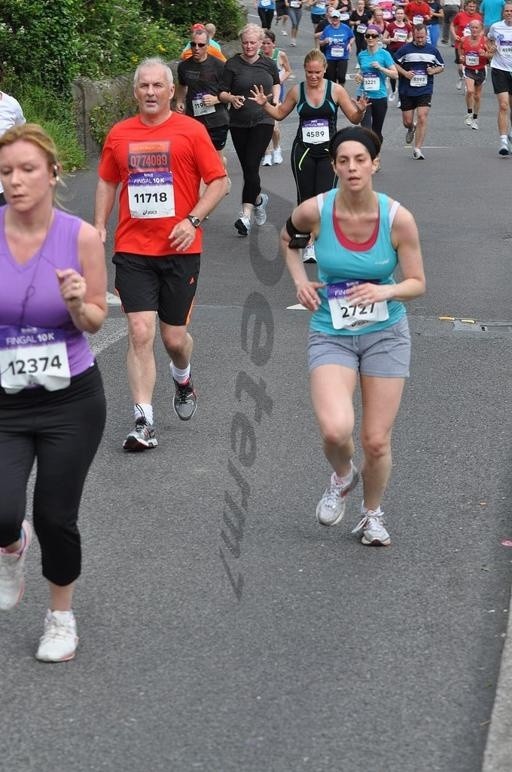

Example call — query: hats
[331,10,340,17]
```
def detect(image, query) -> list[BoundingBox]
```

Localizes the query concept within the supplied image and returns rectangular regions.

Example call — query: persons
[0,83,27,212]
[0,122,108,663]
[176,1,512,265]
[282,127,426,547]
[92,59,232,451]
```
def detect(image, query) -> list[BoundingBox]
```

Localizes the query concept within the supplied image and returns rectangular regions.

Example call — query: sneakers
[124,404,158,450]
[174,372,197,421]
[406,123,417,143]
[316,467,359,526]
[273,147,282,164]
[456,78,463,89]
[410,149,424,160]
[303,246,317,263]
[36,610,77,663]
[472,120,478,129]
[351,503,391,546]
[499,139,510,155]
[466,113,473,125]
[389,88,397,101]
[254,194,268,225]
[263,155,272,166]
[1,520,31,612]
[235,217,250,235]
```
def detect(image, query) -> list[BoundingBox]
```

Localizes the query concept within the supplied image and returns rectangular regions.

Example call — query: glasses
[365,34,378,39]
[190,42,206,47]
